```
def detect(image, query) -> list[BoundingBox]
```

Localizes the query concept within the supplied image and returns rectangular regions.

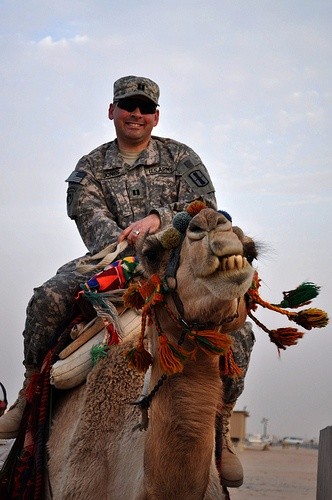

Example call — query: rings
[132,230,141,234]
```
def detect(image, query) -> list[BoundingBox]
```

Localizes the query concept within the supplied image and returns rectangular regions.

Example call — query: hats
[113,76,160,106]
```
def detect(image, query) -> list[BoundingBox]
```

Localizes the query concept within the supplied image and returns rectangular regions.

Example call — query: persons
[0,75,256,487]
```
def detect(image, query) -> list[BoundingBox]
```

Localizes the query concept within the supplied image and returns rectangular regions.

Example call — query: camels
[2,207,258,496]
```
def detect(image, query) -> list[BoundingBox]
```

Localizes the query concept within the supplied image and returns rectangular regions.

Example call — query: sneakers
[217,401,243,487]
[0,367,40,440]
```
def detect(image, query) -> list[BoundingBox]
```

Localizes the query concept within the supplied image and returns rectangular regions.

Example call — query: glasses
[114,98,156,115]
